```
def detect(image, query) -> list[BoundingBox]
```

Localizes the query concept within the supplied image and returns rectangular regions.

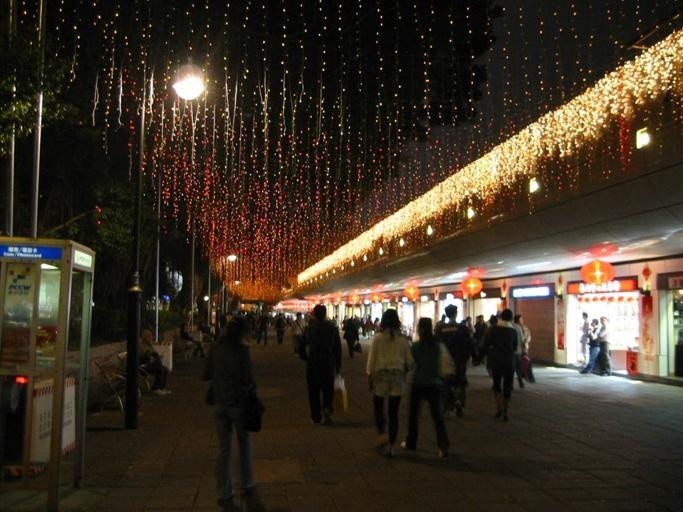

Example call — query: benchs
[92,355,142,414]
[115,351,158,394]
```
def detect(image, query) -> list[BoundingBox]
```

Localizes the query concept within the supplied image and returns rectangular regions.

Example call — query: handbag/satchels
[245,395,264,433]
[438,341,457,379]
[522,355,536,384]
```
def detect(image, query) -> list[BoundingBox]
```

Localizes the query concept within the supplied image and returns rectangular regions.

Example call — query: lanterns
[460,277,483,299]
[577,295,639,303]
[402,285,418,301]
[305,294,382,305]
[578,259,614,288]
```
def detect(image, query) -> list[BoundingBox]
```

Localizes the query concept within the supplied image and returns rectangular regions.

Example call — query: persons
[137,329,172,395]
[578,320,600,374]
[365,309,417,457]
[179,304,532,426]
[578,313,588,364]
[198,320,259,506]
[399,317,452,458]
[591,316,612,377]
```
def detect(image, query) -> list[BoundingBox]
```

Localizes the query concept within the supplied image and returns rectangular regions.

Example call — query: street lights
[214,251,238,337]
[117,57,206,431]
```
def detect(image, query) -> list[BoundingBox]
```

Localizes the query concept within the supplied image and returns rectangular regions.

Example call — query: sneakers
[151,387,172,396]
[215,479,258,500]
[495,407,510,421]
[370,432,416,457]
[311,406,334,426]
[438,446,449,457]
[455,400,464,417]
